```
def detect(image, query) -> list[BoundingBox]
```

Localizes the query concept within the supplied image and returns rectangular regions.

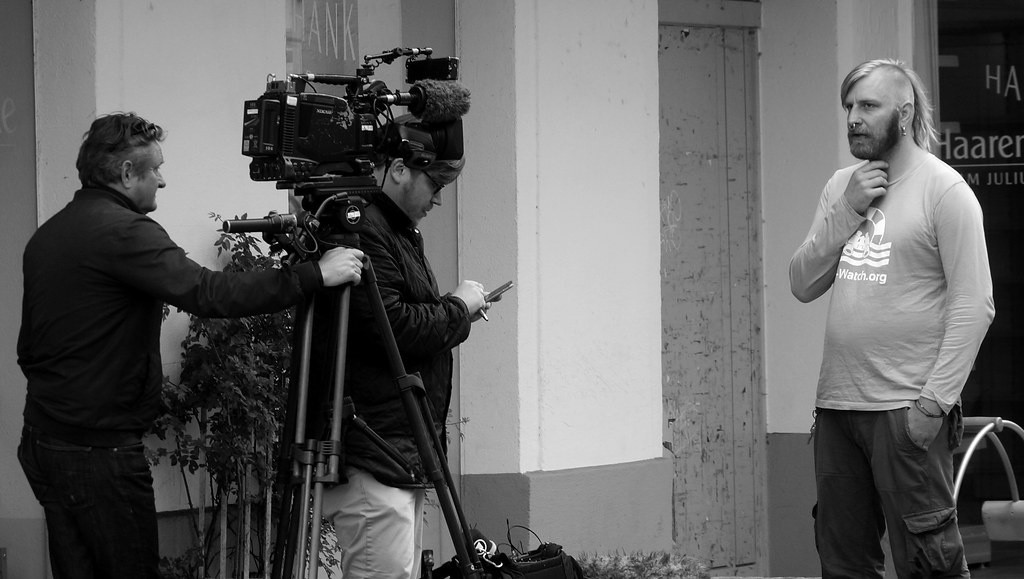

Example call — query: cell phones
[484,280,513,302]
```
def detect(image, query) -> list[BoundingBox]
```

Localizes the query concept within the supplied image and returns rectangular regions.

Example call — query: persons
[287,113,488,578]
[16,110,365,578]
[789,59,995,579]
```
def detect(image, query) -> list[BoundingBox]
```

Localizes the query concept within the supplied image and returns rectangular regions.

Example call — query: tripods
[223,186,475,579]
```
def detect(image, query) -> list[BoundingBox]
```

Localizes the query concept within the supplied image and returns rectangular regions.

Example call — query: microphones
[377,80,472,121]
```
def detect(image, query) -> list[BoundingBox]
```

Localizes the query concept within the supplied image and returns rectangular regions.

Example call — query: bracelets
[916,400,942,419]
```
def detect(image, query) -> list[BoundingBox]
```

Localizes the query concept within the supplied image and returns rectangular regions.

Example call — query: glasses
[424,171,445,196]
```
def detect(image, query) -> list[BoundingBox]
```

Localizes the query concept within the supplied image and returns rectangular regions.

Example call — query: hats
[395,112,465,172]
